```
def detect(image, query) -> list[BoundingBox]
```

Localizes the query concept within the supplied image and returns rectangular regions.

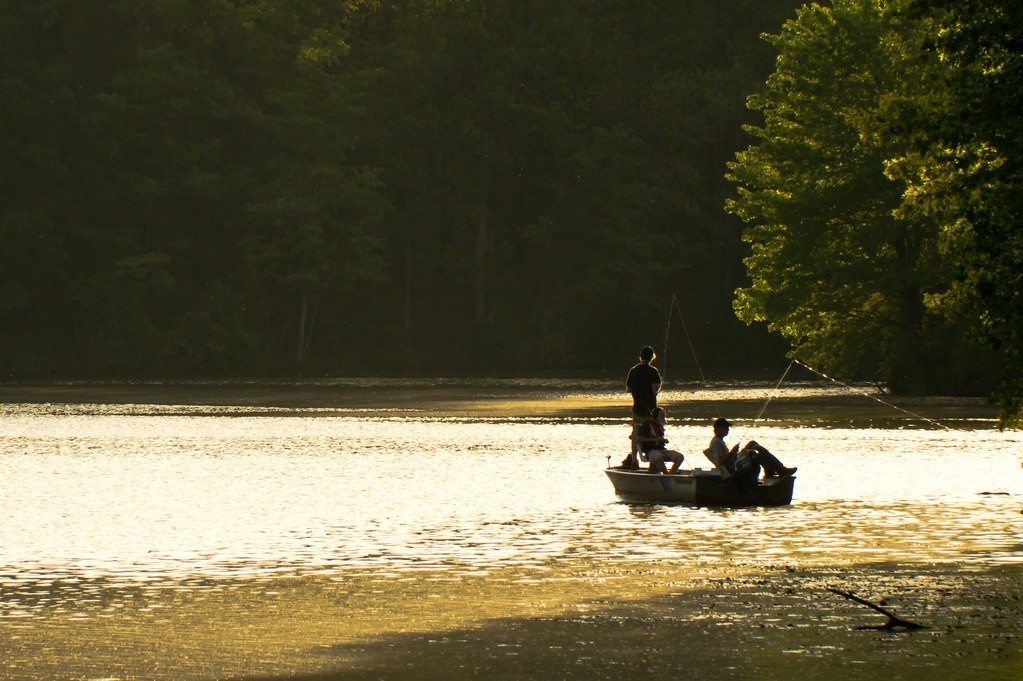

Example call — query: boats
[604,461,794,508]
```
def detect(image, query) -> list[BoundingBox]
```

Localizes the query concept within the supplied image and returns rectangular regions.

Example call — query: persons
[711,417,798,479]
[640,407,685,473]
[626,345,663,467]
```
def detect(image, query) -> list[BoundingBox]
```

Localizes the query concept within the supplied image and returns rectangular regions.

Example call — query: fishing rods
[733,358,797,450]
[658,295,676,380]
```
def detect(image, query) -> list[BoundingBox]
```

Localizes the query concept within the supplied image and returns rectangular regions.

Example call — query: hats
[651,406,667,425]
[640,346,653,358]
[713,418,733,427]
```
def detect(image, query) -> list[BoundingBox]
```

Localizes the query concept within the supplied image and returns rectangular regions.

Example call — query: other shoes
[777,467,797,478]
[765,468,779,477]
[630,460,638,470]
[670,469,681,474]
